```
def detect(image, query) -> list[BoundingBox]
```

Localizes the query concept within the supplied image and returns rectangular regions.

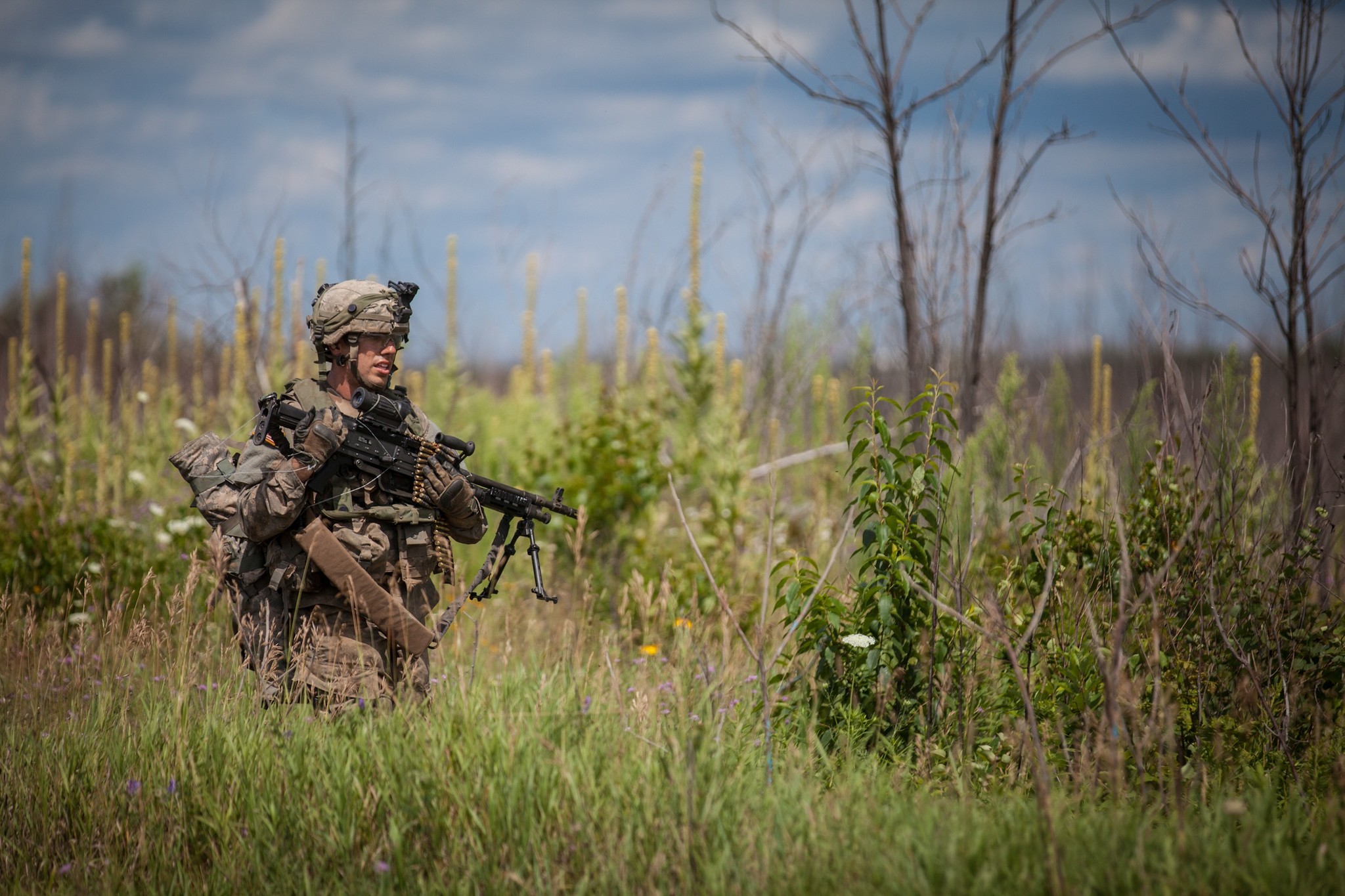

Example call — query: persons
[215,276,488,717]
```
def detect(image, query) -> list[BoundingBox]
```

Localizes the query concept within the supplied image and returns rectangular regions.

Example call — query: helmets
[309,279,420,346]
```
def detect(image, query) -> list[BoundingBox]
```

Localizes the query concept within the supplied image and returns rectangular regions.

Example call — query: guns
[252,384,578,604]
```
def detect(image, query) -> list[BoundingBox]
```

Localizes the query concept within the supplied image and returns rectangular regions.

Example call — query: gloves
[293,405,348,471]
[423,458,484,531]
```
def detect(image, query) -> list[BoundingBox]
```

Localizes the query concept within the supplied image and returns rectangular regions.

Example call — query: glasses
[359,332,402,350]
